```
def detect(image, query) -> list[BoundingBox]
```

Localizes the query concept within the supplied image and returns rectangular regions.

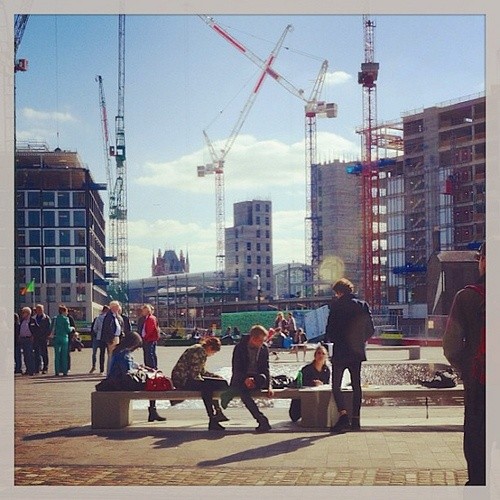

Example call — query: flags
[21,280,34,295]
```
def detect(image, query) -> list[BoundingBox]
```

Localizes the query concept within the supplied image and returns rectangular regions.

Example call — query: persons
[442,240,486,486]
[223,326,241,336]
[172,329,179,336]
[220,325,274,432]
[171,337,228,430]
[263,310,310,354]
[289,346,330,423]
[325,278,375,432]
[90,300,161,375]
[192,329,199,337]
[109,332,185,422]
[206,326,215,337]
[14,301,77,377]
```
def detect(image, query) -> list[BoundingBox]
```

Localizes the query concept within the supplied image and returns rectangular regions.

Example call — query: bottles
[297,370,303,385]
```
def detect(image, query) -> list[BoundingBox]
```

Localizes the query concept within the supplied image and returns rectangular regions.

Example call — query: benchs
[90,382,464,430]
[268,348,319,362]
[366,344,421,360]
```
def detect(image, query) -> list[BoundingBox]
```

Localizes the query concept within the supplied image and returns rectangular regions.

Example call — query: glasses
[475,253,484,260]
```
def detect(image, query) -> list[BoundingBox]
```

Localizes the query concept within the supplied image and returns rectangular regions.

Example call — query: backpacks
[426,368,458,387]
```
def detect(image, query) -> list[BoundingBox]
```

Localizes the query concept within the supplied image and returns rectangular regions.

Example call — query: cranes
[94,14,380,313]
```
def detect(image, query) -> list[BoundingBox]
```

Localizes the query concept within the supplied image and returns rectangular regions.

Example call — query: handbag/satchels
[48,316,57,339]
[145,369,174,390]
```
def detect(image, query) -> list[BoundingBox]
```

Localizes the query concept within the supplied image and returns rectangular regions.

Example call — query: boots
[149,407,164,422]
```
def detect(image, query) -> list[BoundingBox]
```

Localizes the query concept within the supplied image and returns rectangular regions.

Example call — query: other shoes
[256,422,271,430]
[209,423,225,430]
[332,416,349,433]
[350,417,360,430]
[221,391,231,408]
